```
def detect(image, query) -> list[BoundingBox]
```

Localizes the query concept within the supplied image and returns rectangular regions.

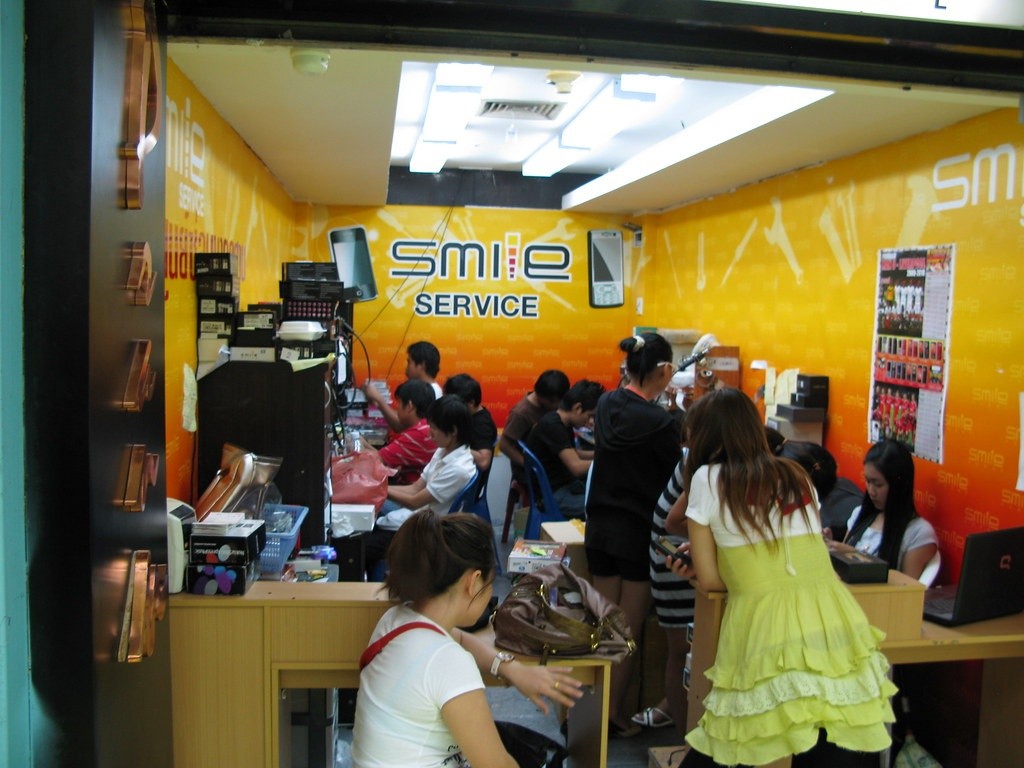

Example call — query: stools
[501,477,530,544]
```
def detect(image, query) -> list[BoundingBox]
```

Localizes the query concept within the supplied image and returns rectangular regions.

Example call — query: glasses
[657,362,678,374]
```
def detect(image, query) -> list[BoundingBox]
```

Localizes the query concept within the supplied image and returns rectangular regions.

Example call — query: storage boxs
[540,518,591,582]
[259,502,308,571]
[184,503,266,597]
[195,252,337,363]
[776,374,829,422]
[345,378,394,428]
[507,540,567,574]
[331,504,376,532]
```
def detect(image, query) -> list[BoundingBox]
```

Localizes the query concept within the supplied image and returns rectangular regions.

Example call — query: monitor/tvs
[335,339,347,385]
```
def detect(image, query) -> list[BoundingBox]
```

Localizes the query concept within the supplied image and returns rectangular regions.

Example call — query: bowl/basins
[278,321,328,341]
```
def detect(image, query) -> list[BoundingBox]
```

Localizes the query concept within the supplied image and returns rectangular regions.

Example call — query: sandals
[631,707,672,726]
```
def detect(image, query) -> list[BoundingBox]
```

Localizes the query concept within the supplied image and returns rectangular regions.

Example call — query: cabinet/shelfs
[195,361,338,566]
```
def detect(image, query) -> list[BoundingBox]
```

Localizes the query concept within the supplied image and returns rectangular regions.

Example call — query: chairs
[880,551,942,768]
[371,435,502,582]
[517,439,566,540]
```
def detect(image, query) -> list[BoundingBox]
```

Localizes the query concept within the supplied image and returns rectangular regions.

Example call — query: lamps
[521,74,685,177]
[409,63,495,173]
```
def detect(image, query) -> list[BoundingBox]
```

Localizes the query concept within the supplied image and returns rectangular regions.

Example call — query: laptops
[922,527,1024,628]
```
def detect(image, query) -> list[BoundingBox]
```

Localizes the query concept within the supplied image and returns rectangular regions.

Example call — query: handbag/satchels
[443,721,568,768]
[491,561,637,663]
[331,452,390,512]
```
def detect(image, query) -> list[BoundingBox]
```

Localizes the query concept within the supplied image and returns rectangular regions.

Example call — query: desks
[170,581,611,768]
[688,566,1024,768]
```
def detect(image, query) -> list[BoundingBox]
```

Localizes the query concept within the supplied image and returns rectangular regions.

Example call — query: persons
[666,387,900,768]
[339,332,938,738]
[349,501,584,768]
[870,277,943,445]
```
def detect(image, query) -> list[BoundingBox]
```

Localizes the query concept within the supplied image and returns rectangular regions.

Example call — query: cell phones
[587,230,625,308]
[328,225,379,302]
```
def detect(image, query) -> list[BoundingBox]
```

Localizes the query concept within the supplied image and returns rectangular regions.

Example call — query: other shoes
[609,721,641,737]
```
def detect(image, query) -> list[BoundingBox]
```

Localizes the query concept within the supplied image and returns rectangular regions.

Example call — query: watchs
[490,650,515,680]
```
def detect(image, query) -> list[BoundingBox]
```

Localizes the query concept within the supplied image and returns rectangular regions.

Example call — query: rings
[554,681,560,688]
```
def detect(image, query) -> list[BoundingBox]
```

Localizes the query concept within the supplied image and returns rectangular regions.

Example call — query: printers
[168,496,197,594]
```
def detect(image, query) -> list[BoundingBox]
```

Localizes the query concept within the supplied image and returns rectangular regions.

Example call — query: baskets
[256,505,309,572]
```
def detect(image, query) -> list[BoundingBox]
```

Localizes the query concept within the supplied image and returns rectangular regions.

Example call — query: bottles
[342,431,365,459]
